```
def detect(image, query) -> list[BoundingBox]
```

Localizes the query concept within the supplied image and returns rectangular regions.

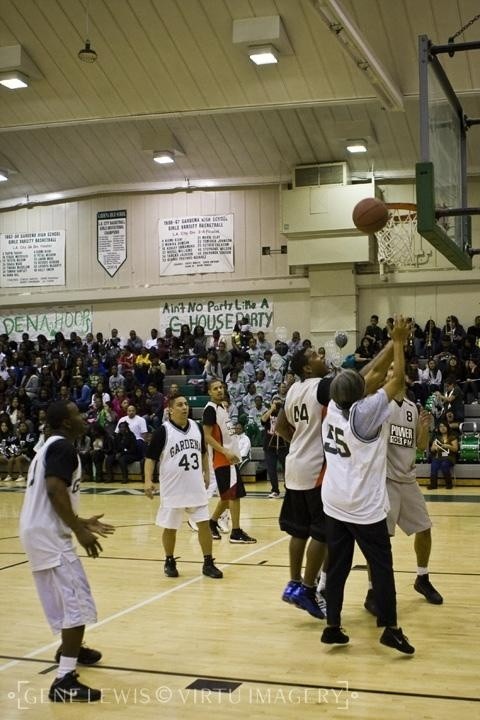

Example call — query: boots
[444,473,453,489]
[92,454,104,482]
[428,474,438,489]
[120,463,128,483]
[104,464,113,482]
[78,451,93,482]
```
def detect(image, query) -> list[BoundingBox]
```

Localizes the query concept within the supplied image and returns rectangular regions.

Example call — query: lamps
[345,140,368,154]
[247,43,280,68]
[0,69,30,94]
[152,151,176,166]
[0,168,9,185]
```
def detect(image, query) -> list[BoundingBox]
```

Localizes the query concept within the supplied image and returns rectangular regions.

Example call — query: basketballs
[352,198,387,232]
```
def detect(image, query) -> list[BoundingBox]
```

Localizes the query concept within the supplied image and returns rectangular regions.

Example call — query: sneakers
[229,529,257,544]
[282,581,302,602]
[315,590,327,616]
[15,475,25,482]
[414,574,442,604]
[49,669,101,702]
[289,584,325,619]
[364,589,377,616]
[4,475,13,481]
[380,626,414,654]
[55,641,101,664]
[269,492,280,499]
[203,558,222,578]
[187,518,199,532]
[321,625,349,643]
[219,516,230,533]
[209,519,223,539]
[164,557,180,577]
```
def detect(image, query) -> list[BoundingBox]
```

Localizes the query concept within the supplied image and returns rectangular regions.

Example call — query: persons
[19,401,116,703]
[202,379,257,544]
[316,547,328,600]
[187,400,230,534]
[0,316,480,500]
[275,340,395,619]
[365,360,443,617]
[143,393,223,579]
[320,311,416,654]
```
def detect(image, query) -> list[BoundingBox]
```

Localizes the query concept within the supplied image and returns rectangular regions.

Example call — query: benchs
[457,403,480,462]
[100,367,271,483]
